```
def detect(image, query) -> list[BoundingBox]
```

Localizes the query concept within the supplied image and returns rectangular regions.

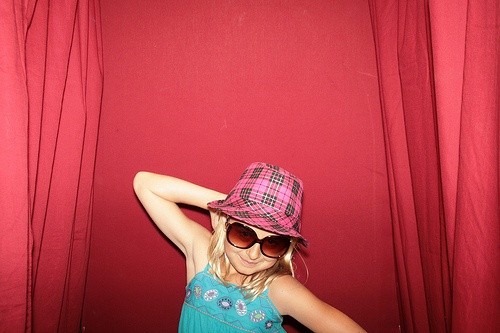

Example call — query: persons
[132,162,366,333]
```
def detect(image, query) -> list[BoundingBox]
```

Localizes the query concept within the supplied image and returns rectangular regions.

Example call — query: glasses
[226,214,291,258]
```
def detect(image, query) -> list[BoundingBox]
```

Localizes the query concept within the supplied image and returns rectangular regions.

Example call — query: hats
[207,161,309,248]
